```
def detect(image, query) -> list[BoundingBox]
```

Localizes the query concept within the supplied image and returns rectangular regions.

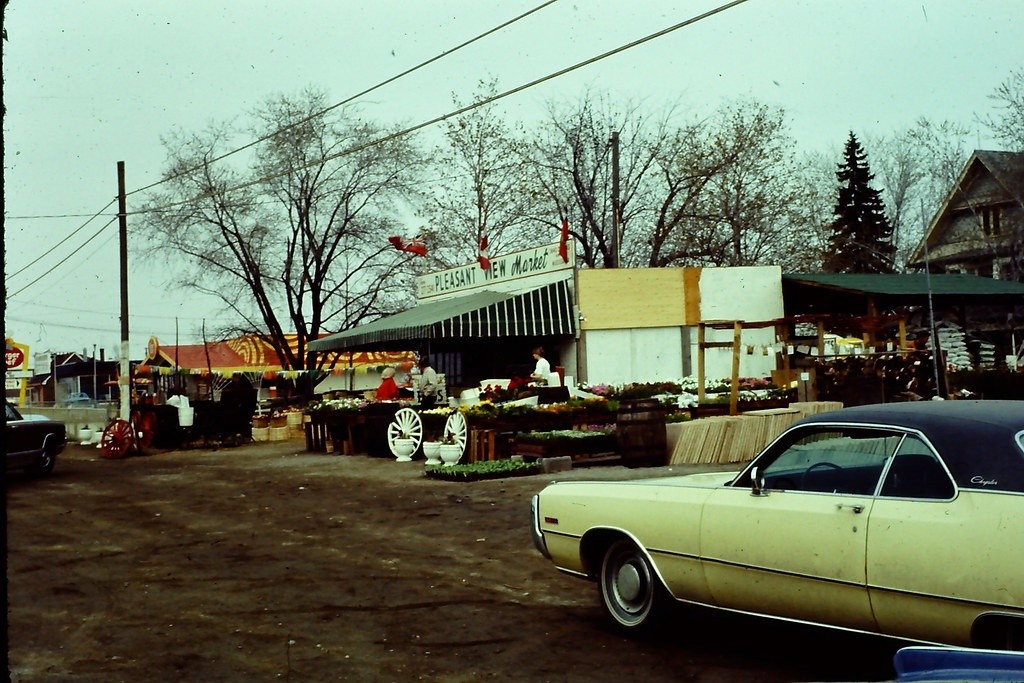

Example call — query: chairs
[865,453,940,500]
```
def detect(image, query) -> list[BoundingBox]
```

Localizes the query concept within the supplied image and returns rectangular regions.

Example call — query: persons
[530,347,551,386]
[377,368,399,400]
[417,356,437,411]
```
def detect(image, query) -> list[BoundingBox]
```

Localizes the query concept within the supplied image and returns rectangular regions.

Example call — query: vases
[270,415,287,428]
[251,414,268,427]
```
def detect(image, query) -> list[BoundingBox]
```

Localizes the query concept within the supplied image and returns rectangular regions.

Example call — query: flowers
[252,375,799,438]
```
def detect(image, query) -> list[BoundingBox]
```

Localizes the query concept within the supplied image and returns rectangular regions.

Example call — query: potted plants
[440,431,460,466]
[78,424,92,445]
[95,428,106,448]
[423,433,443,465]
[394,430,413,462]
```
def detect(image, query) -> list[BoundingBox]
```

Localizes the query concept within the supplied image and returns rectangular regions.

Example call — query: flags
[388,234,427,256]
[476,228,490,270]
[559,220,568,263]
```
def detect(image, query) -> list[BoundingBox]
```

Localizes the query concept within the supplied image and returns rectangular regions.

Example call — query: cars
[527,397,1024,649]
[5,398,67,478]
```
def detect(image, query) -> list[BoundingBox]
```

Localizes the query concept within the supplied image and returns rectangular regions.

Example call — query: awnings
[307,280,576,350]
